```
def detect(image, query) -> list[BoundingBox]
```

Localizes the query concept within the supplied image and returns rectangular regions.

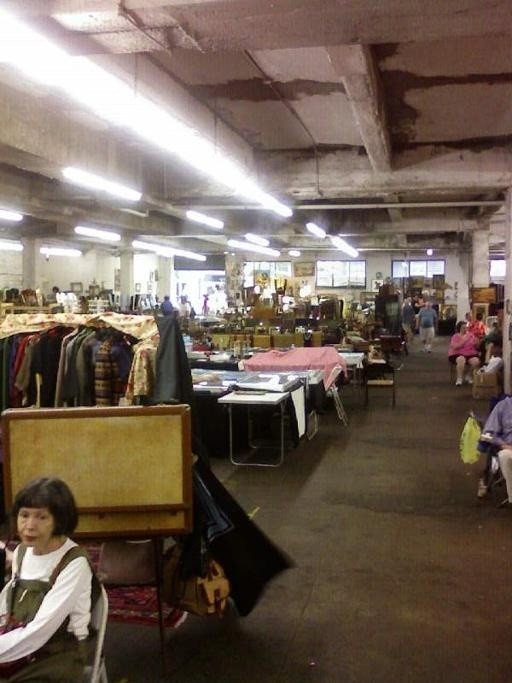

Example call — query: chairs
[477,394,512,507]
[448,331,472,386]
[363,361,396,409]
[80,582,110,683]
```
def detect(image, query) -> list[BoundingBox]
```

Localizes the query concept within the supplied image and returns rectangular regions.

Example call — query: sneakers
[456,376,473,386]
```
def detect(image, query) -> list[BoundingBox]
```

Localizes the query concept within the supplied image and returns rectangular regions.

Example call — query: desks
[185,345,365,468]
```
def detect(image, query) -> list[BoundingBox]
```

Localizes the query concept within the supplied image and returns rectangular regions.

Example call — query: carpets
[6,540,189,628]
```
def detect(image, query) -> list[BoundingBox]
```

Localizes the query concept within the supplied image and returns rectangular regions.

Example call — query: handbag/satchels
[160,545,230,615]
[0,625,34,679]
[96,539,158,586]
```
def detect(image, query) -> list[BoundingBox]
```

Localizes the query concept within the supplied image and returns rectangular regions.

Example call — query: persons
[160,294,174,315]
[1,477,100,682]
[468,313,490,336]
[448,320,481,386]
[176,294,191,319]
[484,395,512,507]
[482,313,504,354]
[465,312,474,334]
[201,293,211,315]
[400,295,438,353]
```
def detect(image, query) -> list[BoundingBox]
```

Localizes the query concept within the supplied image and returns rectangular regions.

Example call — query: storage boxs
[472,368,501,400]
